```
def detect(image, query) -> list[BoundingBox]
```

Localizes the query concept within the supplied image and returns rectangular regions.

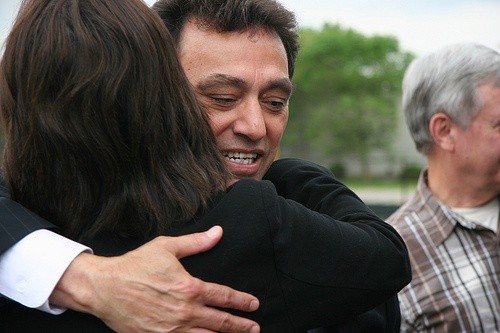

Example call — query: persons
[384,43,500,333]
[0,0,401,333]
[0,0,411,333]
